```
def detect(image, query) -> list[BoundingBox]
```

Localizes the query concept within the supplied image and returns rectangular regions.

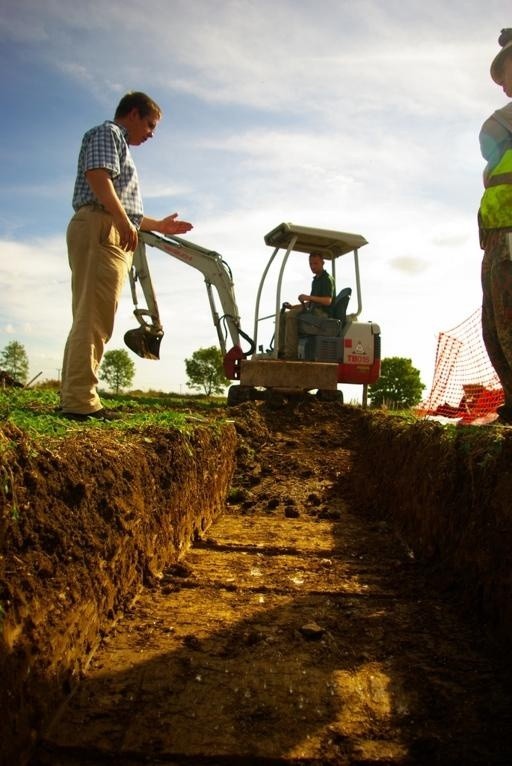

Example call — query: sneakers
[63,409,122,420]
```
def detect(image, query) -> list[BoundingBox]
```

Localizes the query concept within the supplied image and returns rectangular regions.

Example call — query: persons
[276,253,335,361]
[62,90,196,419]
[478,29,511,426]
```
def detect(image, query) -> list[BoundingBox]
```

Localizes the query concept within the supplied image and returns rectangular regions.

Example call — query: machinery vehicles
[122,222,383,408]
